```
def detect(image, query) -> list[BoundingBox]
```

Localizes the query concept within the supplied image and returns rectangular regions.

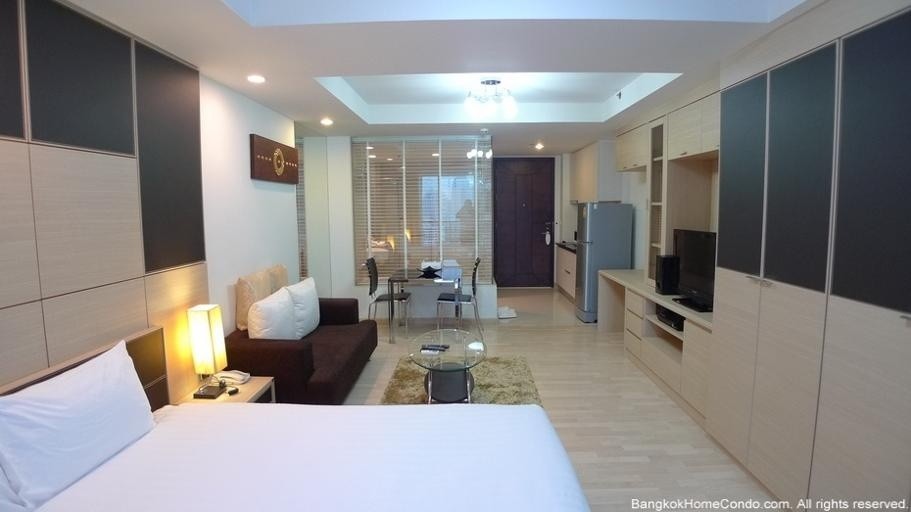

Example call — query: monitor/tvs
[672,229,716,312]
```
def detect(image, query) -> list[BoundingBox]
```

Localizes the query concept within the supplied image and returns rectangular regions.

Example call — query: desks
[389,267,460,343]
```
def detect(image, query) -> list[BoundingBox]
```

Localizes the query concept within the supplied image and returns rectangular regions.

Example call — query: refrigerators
[575,202,631,323]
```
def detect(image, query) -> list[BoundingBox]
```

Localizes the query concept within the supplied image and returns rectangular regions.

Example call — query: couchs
[225,297,377,404]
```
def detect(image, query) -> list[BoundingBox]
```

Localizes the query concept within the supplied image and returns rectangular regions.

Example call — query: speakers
[655,255,685,295]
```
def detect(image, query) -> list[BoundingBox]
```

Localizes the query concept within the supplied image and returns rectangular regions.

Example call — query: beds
[0,327,590,508]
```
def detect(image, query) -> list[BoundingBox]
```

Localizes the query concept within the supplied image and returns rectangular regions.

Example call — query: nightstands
[181,377,277,402]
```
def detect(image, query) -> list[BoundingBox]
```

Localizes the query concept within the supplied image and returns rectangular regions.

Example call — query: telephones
[208,369,250,385]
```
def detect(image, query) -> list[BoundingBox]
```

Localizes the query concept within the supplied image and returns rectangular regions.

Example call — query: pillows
[234,270,270,329]
[287,276,321,339]
[262,264,288,295]
[0,340,157,510]
[250,287,297,340]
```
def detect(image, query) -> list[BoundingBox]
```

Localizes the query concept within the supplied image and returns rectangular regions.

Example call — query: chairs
[436,259,484,343]
[366,256,410,337]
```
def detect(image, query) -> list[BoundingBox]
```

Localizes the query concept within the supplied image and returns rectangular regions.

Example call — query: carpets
[381,353,541,405]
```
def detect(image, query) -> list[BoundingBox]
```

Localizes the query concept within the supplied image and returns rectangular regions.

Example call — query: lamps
[188,304,229,379]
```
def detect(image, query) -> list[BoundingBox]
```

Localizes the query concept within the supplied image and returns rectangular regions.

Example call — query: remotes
[421,350,439,355]
[420,348,445,352]
[422,344,449,349]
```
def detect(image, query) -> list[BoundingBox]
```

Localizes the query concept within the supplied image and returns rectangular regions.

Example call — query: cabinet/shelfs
[556,241,577,304]
[597,265,712,433]
[806,0,910,512]
[668,90,719,160]
[615,118,648,172]
[709,0,839,512]
[644,102,667,291]
[570,140,625,202]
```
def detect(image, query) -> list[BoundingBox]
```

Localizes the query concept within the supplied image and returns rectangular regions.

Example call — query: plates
[416,265,441,275]
